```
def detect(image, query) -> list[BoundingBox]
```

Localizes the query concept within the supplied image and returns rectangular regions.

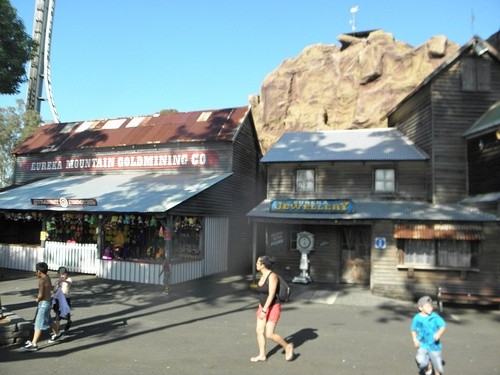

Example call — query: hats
[417,295,433,305]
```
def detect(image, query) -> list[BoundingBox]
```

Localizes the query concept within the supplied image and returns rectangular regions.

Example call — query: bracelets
[262,310,266,313]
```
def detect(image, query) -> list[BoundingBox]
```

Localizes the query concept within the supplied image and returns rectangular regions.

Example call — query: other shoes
[286,342,294,361]
[21,344,37,351]
[48,332,63,343]
[65,320,72,331]
[251,356,267,362]
[424,365,432,375]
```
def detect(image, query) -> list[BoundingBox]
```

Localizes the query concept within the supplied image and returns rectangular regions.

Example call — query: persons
[249,256,293,362]
[102,244,114,261]
[51,267,72,332]
[159,255,171,295]
[410,296,447,375]
[20,262,64,351]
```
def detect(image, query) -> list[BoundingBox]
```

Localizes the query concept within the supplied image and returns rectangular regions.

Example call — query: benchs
[436,282,500,312]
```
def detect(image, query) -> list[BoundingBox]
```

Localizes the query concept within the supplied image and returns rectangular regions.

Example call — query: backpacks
[276,273,290,302]
